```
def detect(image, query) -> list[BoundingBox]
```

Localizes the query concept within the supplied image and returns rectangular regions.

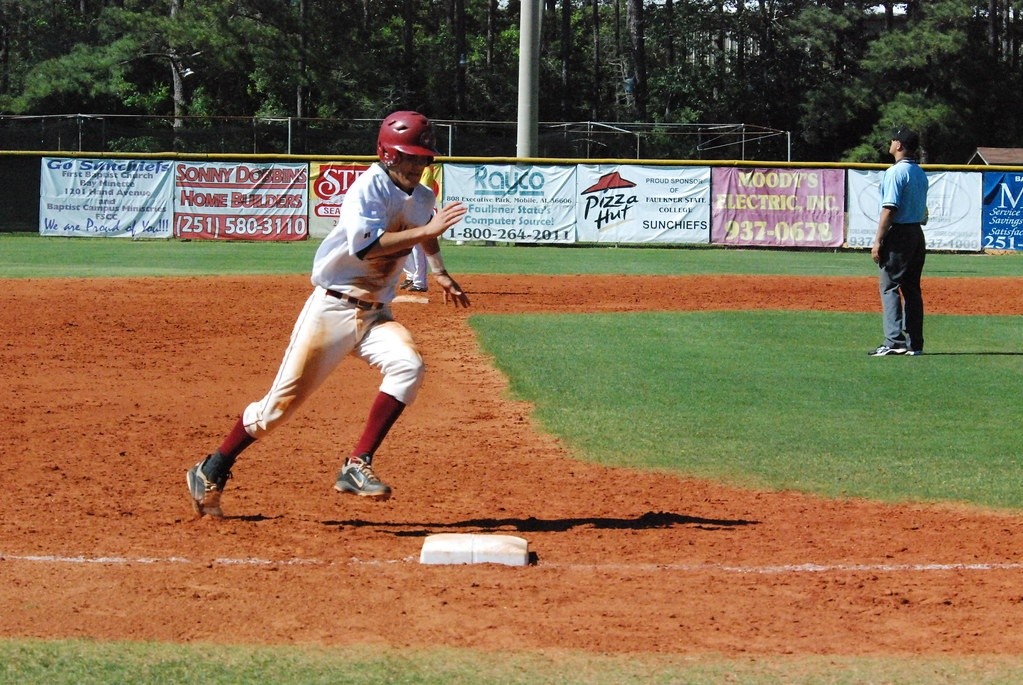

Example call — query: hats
[892,128,919,151]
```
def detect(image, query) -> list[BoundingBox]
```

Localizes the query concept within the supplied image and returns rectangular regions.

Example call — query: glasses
[400,153,434,168]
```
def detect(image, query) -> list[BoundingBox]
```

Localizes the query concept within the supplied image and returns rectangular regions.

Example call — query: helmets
[377,111,437,169]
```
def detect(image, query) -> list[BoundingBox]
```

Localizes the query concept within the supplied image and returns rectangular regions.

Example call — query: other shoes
[409,285,428,292]
[400,280,413,290]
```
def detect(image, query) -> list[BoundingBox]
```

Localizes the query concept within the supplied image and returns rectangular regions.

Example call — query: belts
[326,291,384,311]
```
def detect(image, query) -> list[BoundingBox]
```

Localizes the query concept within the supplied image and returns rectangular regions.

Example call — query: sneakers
[335,454,391,498]
[906,349,922,356]
[868,344,907,356]
[186,453,234,518]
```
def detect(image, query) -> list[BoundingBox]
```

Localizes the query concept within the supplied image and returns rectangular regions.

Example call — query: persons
[186,110,471,516]
[868,129,928,356]
[400,167,433,292]
